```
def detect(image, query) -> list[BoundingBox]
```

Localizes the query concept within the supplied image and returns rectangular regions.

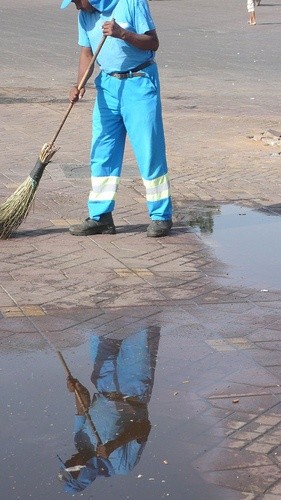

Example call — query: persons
[57,325,161,494]
[61,0,173,237]
[247,0,257,25]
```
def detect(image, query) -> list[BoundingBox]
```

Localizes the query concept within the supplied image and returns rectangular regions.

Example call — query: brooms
[1,18,119,242]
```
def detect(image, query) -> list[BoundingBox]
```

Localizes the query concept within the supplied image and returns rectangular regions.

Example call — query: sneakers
[147,220,172,237]
[69,215,116,235]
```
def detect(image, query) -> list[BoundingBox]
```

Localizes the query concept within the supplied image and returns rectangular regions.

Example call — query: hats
[60,0,119,13]
[57,454,104,495]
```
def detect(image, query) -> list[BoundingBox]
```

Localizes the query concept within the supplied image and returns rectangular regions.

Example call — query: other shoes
[248,21,255,25]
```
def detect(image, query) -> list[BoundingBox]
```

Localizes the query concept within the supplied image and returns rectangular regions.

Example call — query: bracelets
[121,30,125,40]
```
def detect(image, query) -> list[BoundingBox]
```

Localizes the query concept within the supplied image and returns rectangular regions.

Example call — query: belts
[107,59,154,78]
[100,393,148,406]
